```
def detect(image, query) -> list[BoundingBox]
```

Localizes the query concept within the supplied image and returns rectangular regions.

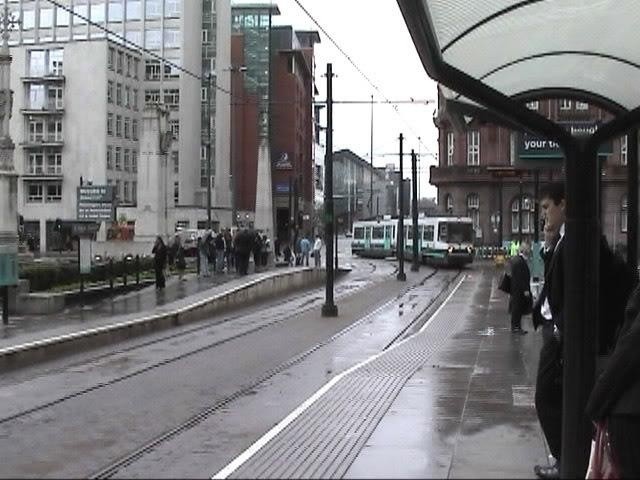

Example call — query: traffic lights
[298,211,303,229]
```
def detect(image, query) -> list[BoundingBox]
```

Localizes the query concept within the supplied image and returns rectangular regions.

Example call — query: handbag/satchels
[497,270,513,292]
[584,421,622,480]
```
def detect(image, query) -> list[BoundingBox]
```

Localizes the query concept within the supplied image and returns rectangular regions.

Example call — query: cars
[169,229,226,258]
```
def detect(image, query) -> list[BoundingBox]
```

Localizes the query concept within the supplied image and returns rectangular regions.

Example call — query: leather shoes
[511,324,529,335]
[533,460,559,479]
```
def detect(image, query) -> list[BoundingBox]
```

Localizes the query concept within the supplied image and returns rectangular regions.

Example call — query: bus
[351,212,475,267]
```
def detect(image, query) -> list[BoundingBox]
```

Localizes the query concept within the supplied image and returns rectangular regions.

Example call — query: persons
[509,181,640,480]
[152,223,322,290]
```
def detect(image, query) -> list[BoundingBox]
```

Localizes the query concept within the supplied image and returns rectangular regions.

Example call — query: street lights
[490,213,501,258]
[207,67,248,230]
